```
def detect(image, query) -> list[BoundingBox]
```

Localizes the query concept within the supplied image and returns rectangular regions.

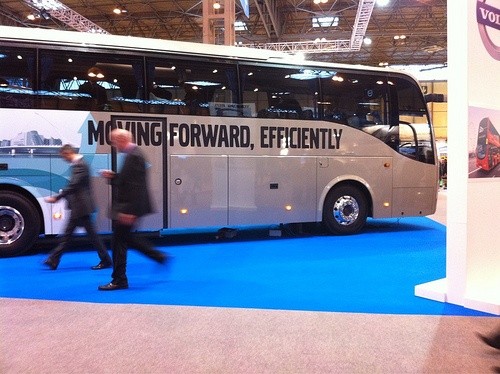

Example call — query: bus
[0,24,444,258]
[475,117,500,171]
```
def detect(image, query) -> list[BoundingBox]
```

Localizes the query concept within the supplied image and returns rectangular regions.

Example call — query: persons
[79,67,303,118]
[42,144,112,270]
[438,157,447,190]
[98,129,171,291]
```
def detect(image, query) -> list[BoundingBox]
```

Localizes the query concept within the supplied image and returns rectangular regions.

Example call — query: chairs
[257,99,314,118]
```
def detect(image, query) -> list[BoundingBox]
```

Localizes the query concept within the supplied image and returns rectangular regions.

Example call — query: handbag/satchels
[116,211,138,227]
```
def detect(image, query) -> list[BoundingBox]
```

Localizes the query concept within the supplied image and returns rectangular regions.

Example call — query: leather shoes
[91,259,113,269]
[43,258,58,271]
[98,278,129,291]
